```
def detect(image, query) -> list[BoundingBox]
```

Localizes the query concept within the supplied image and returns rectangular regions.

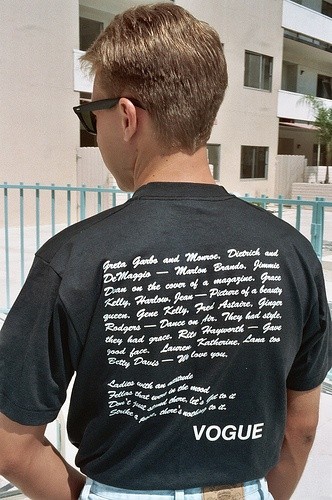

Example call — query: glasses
[73,96,147,136]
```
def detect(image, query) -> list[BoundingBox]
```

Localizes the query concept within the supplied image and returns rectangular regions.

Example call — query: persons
[0,3,332,500]
